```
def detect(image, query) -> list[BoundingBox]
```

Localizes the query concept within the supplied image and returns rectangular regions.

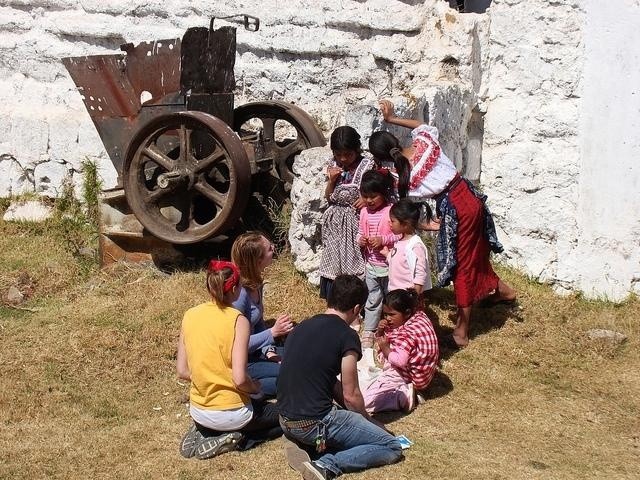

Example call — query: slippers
[479,295,516,308]
[438,334,468,349]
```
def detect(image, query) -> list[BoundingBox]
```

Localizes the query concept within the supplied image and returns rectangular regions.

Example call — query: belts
[278,413,316,430]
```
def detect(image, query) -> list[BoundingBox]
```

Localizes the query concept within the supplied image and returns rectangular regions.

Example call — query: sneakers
[398,382,415,411]
[179,422,245,460]
[281,433,336,480]
